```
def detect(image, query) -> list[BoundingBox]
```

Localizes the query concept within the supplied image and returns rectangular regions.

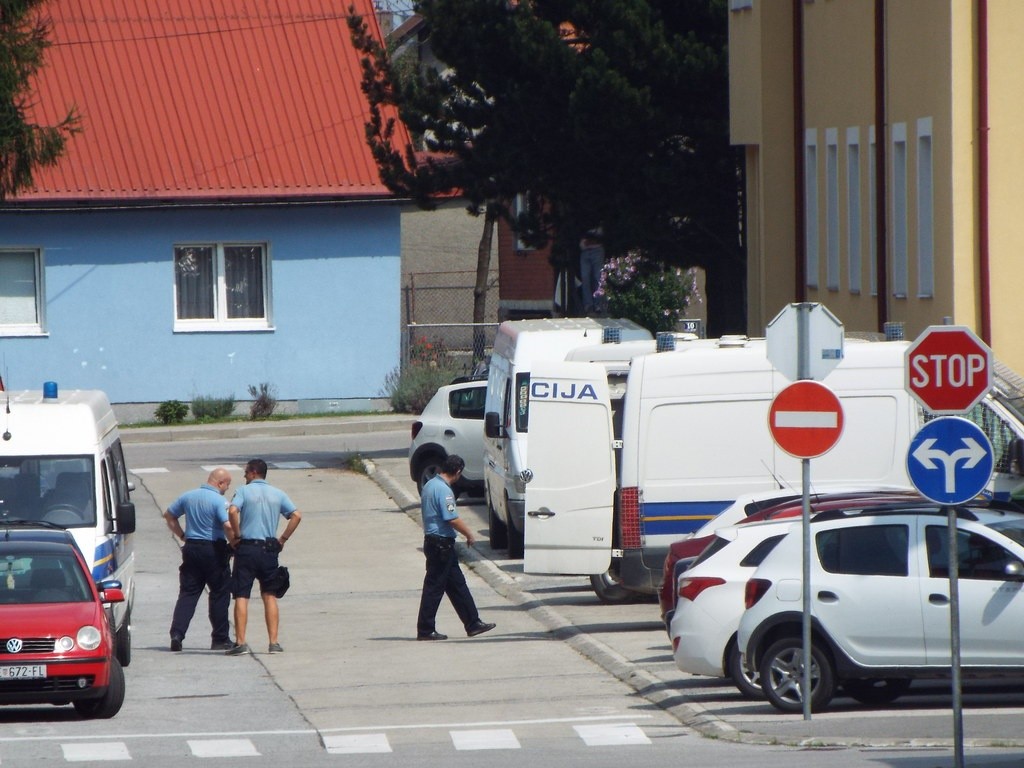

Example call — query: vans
[483,316,658,559]
[0,381,137,667]
[523,334,1024,607]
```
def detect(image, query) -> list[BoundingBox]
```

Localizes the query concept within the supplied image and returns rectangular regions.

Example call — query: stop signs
[901,324,995,416]
[768,379,845,459]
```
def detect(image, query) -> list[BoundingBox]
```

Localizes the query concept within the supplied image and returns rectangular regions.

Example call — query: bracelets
[234,536,241,539]
[281,536,287,540]
[181,532,184,539]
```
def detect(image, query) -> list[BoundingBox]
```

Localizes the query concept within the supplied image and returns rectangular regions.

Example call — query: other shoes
[416,629,447,640]
[211,637,234,649]
[467,620,496,636]
[269,643,283,653]
[225,642,249,655]
[170,631,182,651]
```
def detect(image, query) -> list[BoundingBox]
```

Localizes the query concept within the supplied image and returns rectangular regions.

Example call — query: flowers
[407,333,451,369]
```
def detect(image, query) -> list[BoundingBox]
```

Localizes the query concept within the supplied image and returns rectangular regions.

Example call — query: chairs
[23,558,65,601]
[835,529,967,577]
[0,470,98,522]
[462,396,481,409]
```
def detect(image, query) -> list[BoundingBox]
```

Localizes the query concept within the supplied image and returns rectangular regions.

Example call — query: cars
[409,380,488,501]
[657,480,1024,714]
[0,527,126,720]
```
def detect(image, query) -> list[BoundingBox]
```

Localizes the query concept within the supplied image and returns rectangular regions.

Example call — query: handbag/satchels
[276,565,290,598]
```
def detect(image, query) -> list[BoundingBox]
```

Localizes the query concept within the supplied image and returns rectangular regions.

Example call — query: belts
[240,539,265,546]
[424,535,454,543]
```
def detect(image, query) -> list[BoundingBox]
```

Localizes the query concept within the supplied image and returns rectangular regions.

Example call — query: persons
[163,468,236,650]
[417,455,497,639]
[224,459,302,655]
